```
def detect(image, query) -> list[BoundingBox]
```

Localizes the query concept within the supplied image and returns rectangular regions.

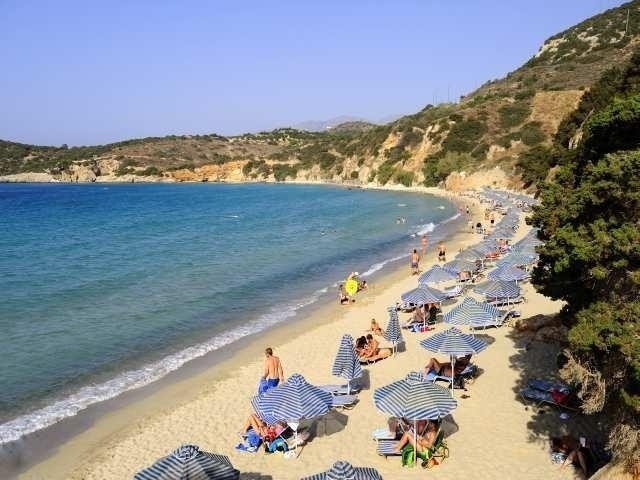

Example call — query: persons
[240,414,286,438]
[354,336,367,355]
[407,306,430,325]
[411,250,419,275]
[394,420,438,452]
[425,357,465,376]
[360,334,379,357]
[388,419,424,435]
[338,271,367,305]
[261,348,284,387]
[427,302,437,322]
[370,319,385,336]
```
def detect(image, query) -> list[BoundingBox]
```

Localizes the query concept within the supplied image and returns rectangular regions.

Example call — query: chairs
[521,377,583,418]
[256,267,516,461]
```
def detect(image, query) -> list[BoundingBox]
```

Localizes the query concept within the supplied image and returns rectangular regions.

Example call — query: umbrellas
[134,444,240,480]
[420,326,489,395]
[331,334,363,395]
[384,305,404,358]
[444,296,511,339]
[399,284,448,331]
[251,373,333,453]
[299,460,381,480]
[417,190,544,308]
[373,372,457,463]
